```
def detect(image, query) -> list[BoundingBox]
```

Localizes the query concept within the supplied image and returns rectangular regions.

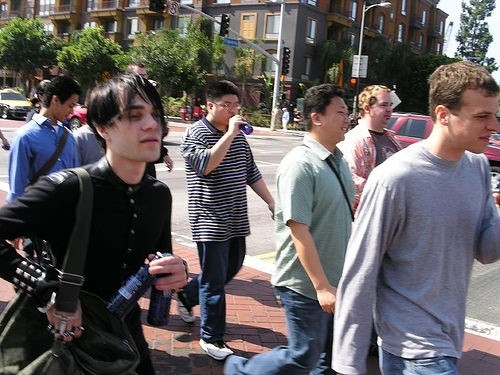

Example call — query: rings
[73,325,85,330]
[63,330,75,336]
[47,323,54,331]
[51,329,59,334]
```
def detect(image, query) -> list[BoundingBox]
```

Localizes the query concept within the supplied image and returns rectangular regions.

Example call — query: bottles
[106,252,164,318]
[147,252,172,327]
[232,115,253,135]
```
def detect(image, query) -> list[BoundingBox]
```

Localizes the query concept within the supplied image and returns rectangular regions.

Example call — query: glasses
[212,101,241,111]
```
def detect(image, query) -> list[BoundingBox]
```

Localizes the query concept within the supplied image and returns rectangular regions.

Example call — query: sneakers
[174,291,195,323]
[199,338,234,360]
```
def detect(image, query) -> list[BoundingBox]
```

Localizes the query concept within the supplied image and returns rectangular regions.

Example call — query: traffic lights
[349,77,358,86]
[281,46,291,76]
[218,13,230,36]
[148,0,167,13]
[279,93,286,100]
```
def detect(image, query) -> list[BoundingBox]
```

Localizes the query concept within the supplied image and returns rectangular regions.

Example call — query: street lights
[350,0,393,128]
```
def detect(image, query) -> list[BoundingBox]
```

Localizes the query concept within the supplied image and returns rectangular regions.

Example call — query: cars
[0,89,40,120]
[66,103,88,132]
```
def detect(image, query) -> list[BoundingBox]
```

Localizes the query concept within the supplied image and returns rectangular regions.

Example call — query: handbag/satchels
[0,280,140,375]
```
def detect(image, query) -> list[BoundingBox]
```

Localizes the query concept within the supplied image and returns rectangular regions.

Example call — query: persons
[25,80,51,124]
[0,130,10,151]
[329,60,500,375]
[0,75,188,375]
[337,84,403,223]
[125,62,172,178]
[281,108,290,130]
[223,85,358,375]
[175,81,276,359]
[293,108,301,130]
[5,75,81,204]
[288,104,295,128]
[72,83,108,168]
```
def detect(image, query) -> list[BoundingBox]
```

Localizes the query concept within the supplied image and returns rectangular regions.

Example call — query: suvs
[385,111,500,194]
